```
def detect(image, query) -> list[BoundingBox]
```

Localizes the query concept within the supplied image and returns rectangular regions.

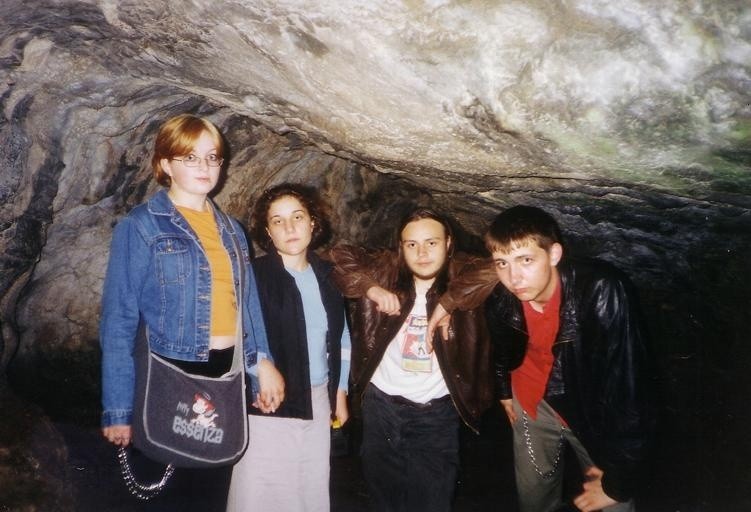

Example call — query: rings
[113,436,121,443]
[121,437,130,443]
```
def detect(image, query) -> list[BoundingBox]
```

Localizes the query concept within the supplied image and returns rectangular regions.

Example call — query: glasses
[170,156,224,167]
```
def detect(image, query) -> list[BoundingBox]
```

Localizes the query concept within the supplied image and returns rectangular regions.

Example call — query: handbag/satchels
[128,356,249,472]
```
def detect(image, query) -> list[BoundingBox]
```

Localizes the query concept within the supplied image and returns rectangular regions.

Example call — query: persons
[99,112,288,512]
[315,204,501,512]
[480,203,662,512]
[225,182,354,512]
